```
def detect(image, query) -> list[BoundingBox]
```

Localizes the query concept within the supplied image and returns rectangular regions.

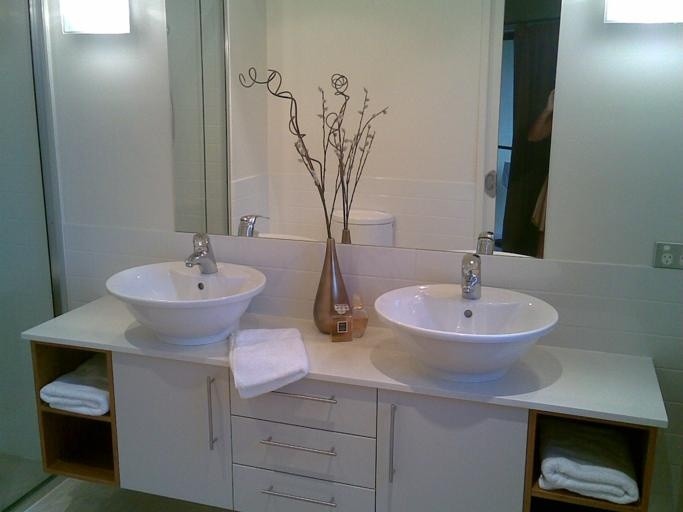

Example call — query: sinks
[105,261,267,346]
[374,284,559,383]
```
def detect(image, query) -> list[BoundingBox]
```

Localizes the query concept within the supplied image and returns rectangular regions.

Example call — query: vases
[313,237,351,333]
[342,230,351,244]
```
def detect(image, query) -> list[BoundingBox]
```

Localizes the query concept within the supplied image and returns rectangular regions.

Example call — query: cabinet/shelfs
[30,339,119,490]
[522,407,659,512]
[376,388,530,512]
[112,351,233,512]
[228,368,378,512]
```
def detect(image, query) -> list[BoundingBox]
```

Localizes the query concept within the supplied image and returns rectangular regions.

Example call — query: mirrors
[165,0,563,260]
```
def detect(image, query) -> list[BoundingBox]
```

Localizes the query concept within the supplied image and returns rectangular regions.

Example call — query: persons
[527,88,555,259]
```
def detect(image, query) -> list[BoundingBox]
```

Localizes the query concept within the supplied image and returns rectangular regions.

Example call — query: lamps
[603,0,683,23]
[62,0,132,34]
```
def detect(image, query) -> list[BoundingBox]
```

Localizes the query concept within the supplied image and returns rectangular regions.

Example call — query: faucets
[185,232,217,274]
[461,254,482,299]
[476,232,495,255]
[237,215,270,236]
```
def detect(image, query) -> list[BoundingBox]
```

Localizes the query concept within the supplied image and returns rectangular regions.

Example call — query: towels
[538,420,640,504]
[229,328,309,399]
[39,353,109,417]
